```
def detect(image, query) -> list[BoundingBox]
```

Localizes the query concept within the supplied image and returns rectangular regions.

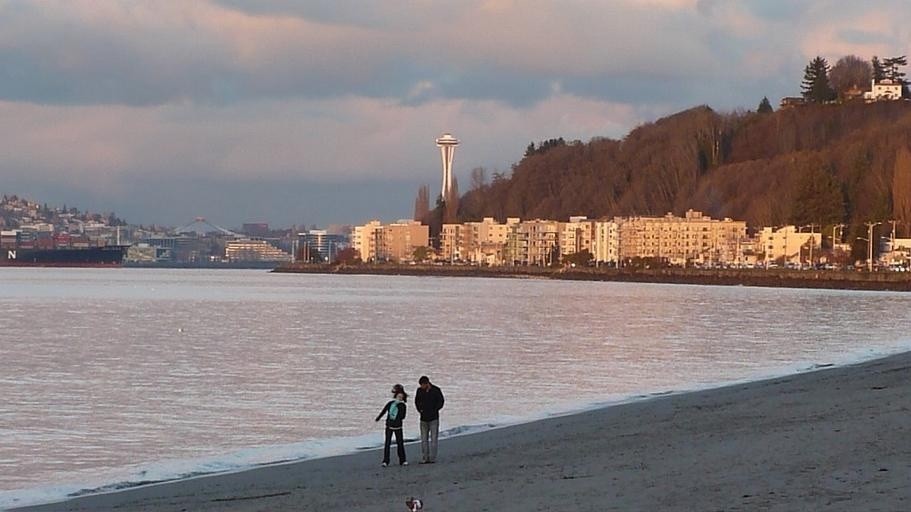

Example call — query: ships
[1,227,130,269]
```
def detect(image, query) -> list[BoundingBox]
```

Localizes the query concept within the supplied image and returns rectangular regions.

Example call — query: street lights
[682,220,910,274]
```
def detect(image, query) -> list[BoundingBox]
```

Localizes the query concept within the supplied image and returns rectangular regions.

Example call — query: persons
[413,376,445,465]
[373,384,410,468]
